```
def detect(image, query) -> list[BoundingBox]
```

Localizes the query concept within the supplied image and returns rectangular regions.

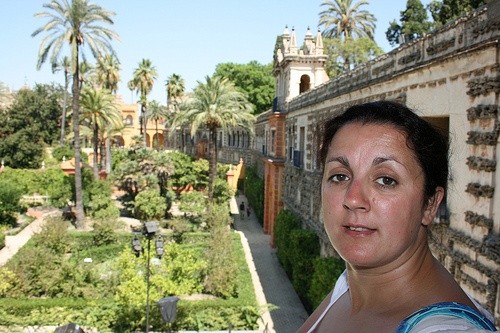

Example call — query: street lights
[132,221,164,333]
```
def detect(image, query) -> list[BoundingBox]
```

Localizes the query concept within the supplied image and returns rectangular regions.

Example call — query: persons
[247,207,252,217]
[239,201,245,220]
[291,101,500,333]
